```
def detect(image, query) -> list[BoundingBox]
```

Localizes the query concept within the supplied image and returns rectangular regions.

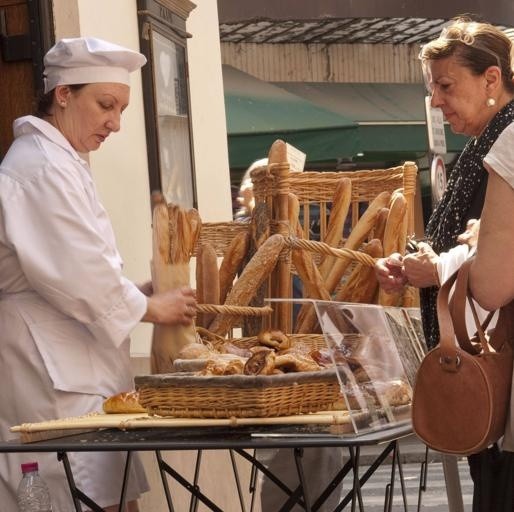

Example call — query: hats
[43,36,147,92]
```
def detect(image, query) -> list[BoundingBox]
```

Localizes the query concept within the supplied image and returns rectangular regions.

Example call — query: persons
[0,34,200,512]
[458,119,514,512]
[376,12,514,512]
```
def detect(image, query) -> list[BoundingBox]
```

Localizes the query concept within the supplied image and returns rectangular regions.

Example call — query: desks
[0,424,429,512]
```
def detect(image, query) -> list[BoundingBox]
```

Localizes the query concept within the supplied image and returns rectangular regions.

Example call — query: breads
[151,177,412,407]
[102,390,149,413]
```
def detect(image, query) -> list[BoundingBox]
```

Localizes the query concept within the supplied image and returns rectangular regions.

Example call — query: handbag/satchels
[412,330,513,458]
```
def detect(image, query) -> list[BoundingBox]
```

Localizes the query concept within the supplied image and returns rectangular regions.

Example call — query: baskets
[133,332,371,418]
